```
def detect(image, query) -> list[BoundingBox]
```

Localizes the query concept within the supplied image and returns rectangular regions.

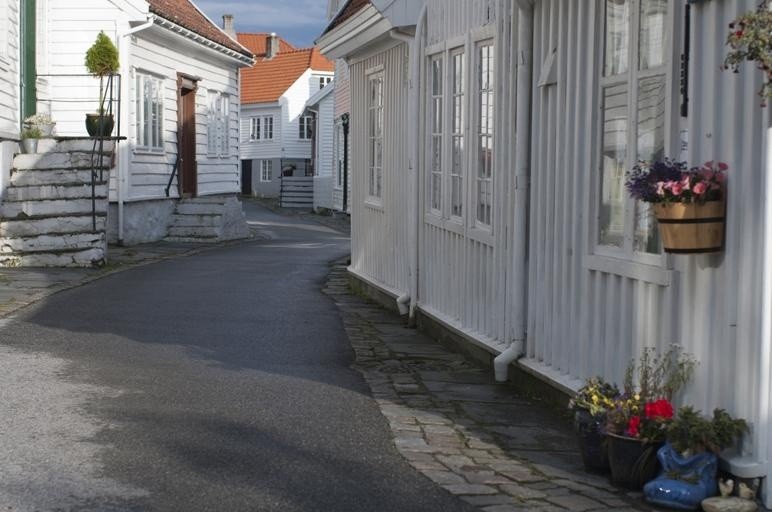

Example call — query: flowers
[624,155,729,211]
[567,341,700,442]
[718,2,772,111]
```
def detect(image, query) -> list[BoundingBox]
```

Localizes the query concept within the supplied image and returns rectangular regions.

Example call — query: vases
[654,200,726,255]
[603,432,664,492]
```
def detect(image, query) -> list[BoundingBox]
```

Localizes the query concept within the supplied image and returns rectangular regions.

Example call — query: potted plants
[644,403,750,511]
[83,29,121,138]
[20,125,42,154]
